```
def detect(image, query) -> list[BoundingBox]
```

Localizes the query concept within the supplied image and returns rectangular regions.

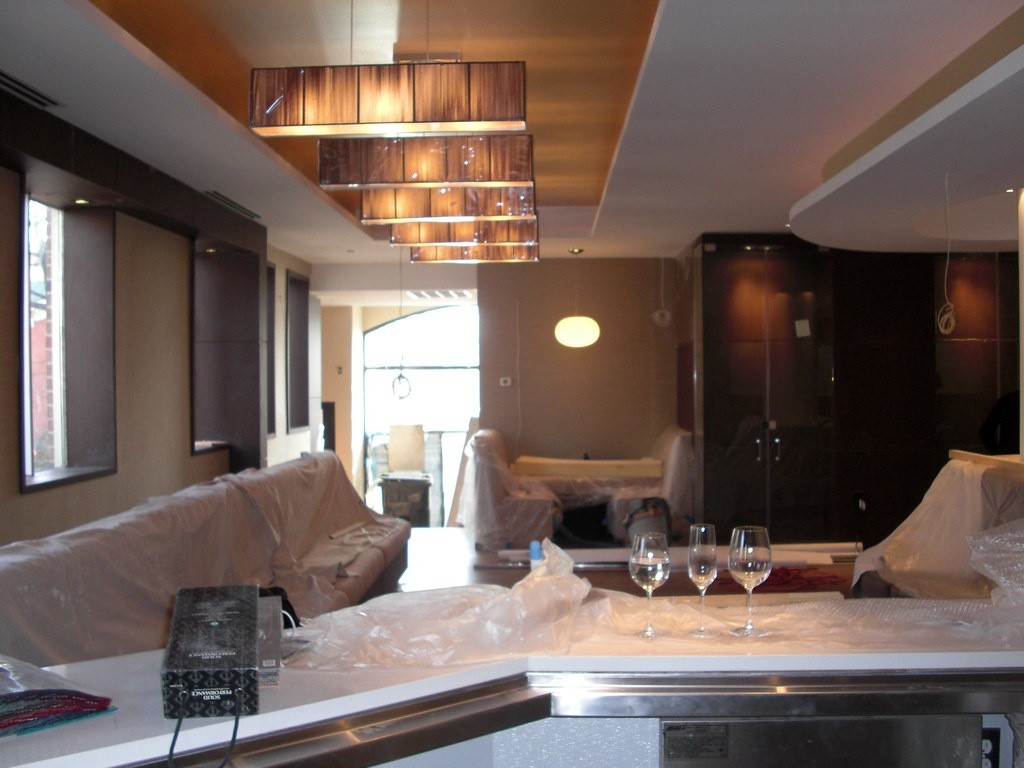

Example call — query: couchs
[0,449,411,666]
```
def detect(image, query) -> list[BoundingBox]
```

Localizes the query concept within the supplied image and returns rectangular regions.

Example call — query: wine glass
[687,524,720,638]
[629,532,671,638]
[728,526,772,638]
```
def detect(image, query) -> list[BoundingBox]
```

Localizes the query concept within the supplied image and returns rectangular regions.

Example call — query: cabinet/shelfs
[693,243,824,545]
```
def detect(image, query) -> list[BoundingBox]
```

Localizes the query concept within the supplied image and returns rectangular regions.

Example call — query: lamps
[554,248,601,349]
[251,1,539,263]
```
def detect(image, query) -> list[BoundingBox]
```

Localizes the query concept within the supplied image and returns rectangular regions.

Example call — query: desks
[513,475,662,497]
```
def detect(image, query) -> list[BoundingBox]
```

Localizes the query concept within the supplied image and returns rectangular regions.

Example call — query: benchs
[610,426,697,542]
[470,430,555,550]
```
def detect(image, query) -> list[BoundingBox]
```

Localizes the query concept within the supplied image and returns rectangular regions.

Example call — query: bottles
[530,540,542,570]
